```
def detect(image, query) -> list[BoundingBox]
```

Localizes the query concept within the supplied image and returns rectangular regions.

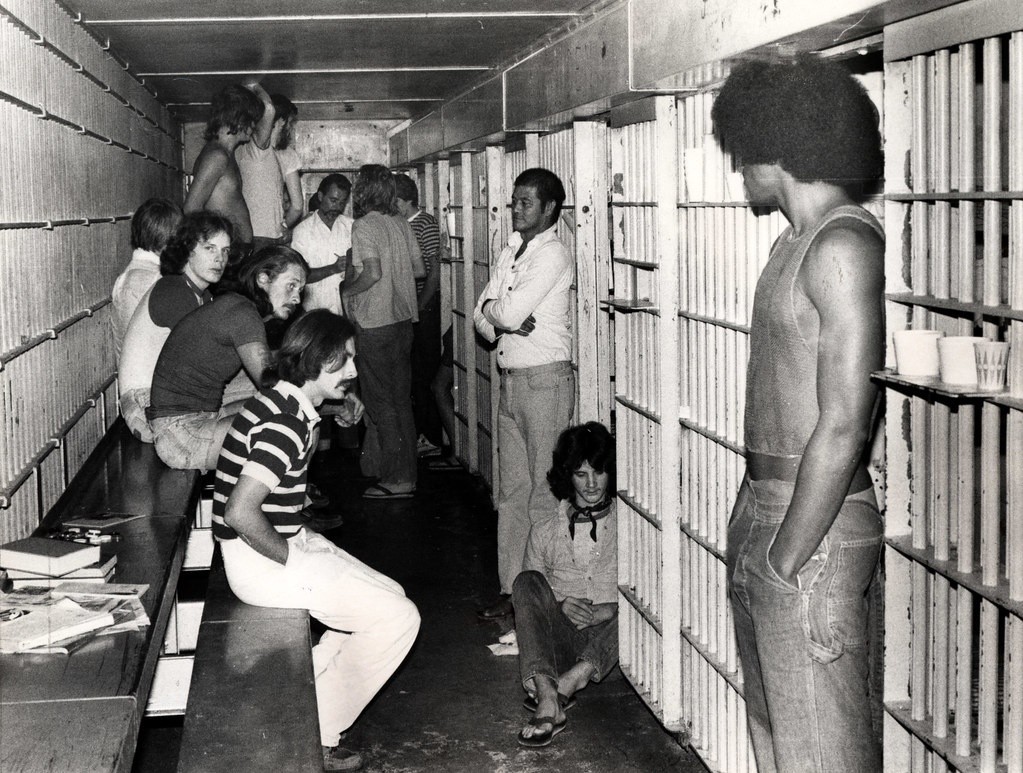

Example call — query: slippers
[361,483,414,499]
[517,695,577,747]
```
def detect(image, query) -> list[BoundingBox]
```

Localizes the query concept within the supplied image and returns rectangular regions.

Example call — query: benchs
[0,415,324,773]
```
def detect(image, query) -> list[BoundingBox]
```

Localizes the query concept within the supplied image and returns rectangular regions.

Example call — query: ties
[568,497,611,542]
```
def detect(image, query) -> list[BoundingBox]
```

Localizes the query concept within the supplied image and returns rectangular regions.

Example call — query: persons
[512,420,619,747]
[109,80,466,533]
[712,51,886,773]
[472,168,575,620]
[212,308,421,771]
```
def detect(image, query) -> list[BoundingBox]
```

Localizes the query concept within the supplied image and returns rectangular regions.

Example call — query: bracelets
[281,221,289,229]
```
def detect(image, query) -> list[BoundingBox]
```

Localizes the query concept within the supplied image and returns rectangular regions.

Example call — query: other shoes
[321,746,362,772]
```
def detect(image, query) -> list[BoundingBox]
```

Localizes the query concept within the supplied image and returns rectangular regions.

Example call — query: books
[0,511,153,656]
